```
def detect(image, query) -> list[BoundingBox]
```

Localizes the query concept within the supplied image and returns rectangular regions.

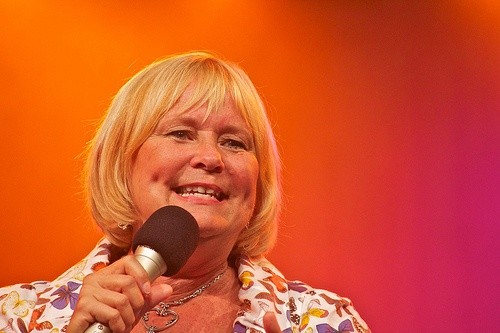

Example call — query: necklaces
[142,267,228,332]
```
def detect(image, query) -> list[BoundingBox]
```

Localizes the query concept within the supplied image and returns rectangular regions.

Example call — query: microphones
[82,206,201,333]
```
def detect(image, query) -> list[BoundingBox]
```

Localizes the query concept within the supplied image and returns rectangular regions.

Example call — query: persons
[1,52,375,333]
